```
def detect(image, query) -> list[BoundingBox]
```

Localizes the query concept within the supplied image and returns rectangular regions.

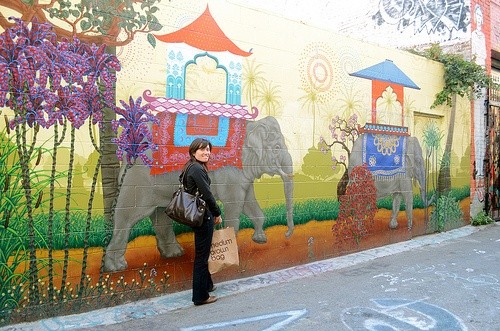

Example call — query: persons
[178,138,222,306]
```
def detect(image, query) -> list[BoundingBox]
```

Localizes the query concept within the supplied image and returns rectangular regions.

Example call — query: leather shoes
[211,285,217,291]
[197,296,217,304]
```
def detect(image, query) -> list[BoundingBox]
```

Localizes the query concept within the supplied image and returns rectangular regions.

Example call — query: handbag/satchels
[164,162,205,227]
[208,221,239,274]
